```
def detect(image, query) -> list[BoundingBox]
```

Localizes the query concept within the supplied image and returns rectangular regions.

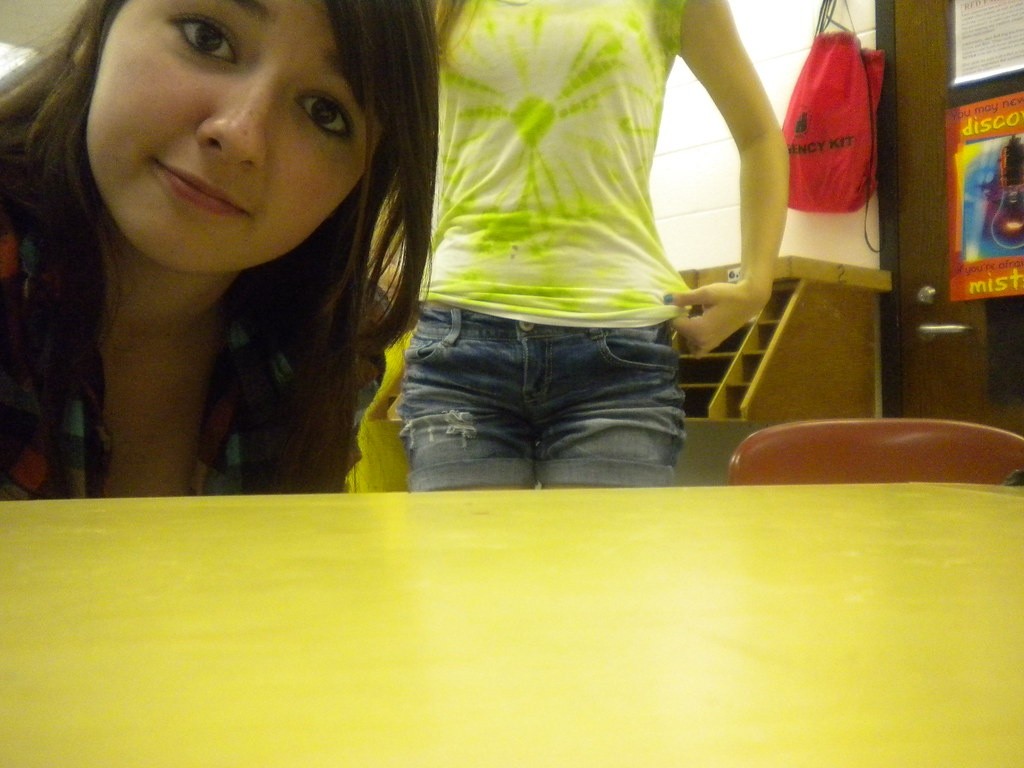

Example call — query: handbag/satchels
[782,0,886,213]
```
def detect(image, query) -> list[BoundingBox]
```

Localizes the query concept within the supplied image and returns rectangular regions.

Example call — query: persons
[0,0,442,499]
[397,0,790,495]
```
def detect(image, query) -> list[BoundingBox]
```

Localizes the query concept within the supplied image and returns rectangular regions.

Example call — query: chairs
[727,416,1024,486]
